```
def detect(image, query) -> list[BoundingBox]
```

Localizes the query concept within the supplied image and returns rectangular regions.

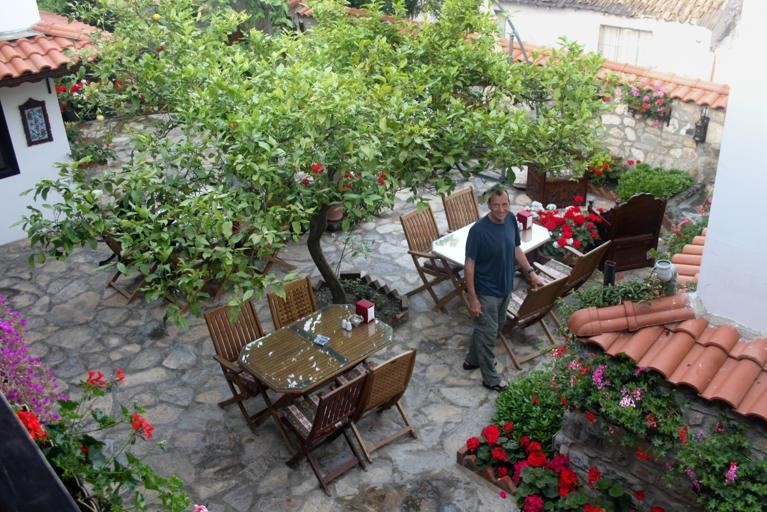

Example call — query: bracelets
[521,268,535,275]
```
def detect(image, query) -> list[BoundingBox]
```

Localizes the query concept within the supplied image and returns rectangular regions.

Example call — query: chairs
[587,193,668,273]
[268,277,315,332]
[356,347,418,463]
[496,274,569,370]
[442,185,480,232]
[533,239,612,340]
[204,299,268,433]
[400,205,462,313]
[285,371,372,496]
[91,204,161,304]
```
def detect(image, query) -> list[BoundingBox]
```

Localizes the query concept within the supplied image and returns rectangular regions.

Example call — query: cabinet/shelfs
[525,163,589,207]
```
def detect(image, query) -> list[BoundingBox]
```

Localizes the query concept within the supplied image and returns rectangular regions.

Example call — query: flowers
[596,78,612,103]
[615,82,670,121]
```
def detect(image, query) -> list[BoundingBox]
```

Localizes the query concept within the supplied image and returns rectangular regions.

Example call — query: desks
[237,304,392,452]
[431,212,553,311]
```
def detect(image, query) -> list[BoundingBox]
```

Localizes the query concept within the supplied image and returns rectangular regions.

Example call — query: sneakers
[463,359,497,370]
[482,379,509,391]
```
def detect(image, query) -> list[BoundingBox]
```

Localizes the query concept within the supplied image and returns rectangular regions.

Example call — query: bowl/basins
[348,314,364,327]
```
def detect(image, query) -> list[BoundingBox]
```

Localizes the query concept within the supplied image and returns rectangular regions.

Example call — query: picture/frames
[17,97,53,146]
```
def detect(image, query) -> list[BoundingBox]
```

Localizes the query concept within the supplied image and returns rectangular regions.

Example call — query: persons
[463,187,544,393]
